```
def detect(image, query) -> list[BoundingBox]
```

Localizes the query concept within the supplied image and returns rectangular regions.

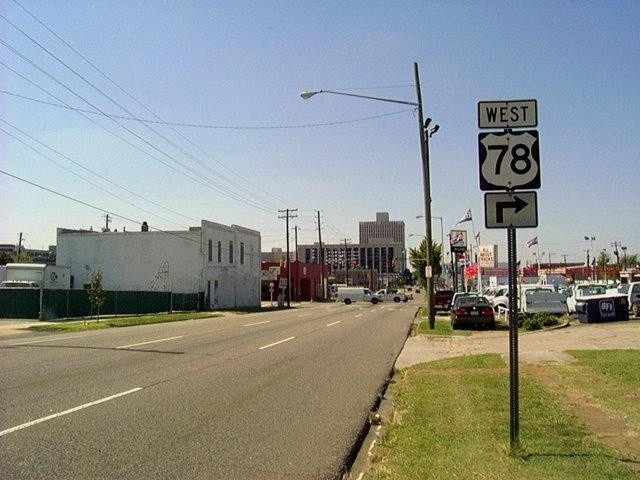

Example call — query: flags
[451,233,463,244]
[455,208,472,226]
[458,253,468,267]
[527,236,538,248]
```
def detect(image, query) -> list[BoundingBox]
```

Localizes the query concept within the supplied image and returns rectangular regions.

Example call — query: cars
[434,280,640,332]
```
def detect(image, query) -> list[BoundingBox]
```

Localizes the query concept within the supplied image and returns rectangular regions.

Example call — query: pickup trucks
[374,288,414,303]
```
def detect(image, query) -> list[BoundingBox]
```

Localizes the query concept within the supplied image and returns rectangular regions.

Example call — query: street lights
[299,60,442,333]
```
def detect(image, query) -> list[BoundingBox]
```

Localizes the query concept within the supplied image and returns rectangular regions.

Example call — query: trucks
[337,285,383,305]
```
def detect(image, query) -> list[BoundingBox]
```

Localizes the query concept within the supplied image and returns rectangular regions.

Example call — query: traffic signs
[476,98,538,129]
[476,131,543,190]
